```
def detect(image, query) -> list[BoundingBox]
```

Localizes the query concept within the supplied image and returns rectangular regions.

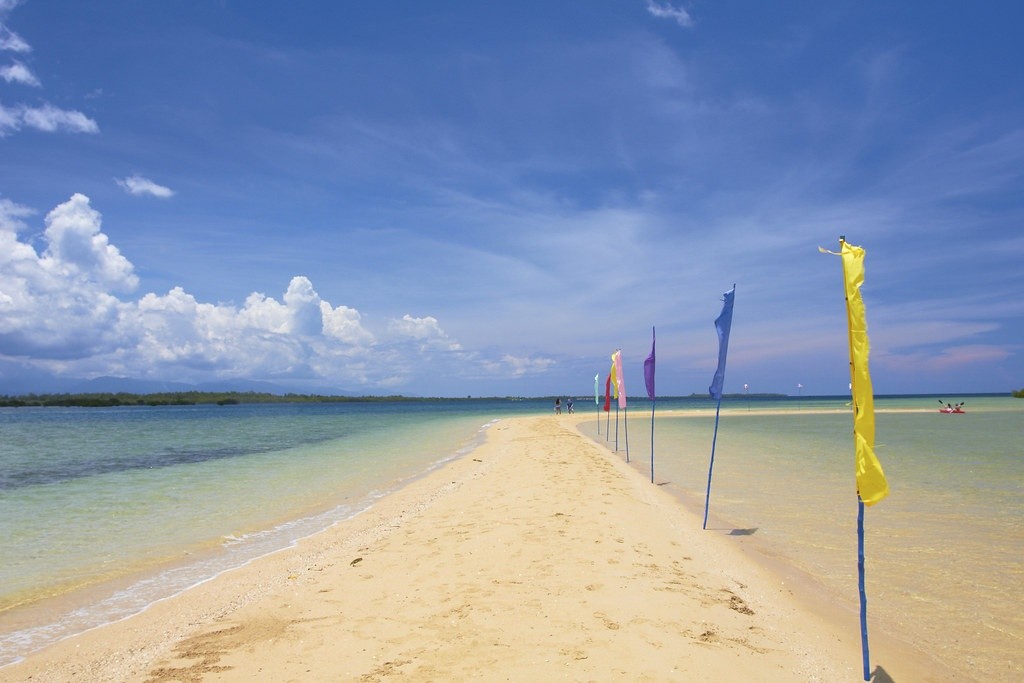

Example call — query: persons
[567,399,574,414]
[945,404,953,410]
[955,403,961,410]
[555,398,561,414]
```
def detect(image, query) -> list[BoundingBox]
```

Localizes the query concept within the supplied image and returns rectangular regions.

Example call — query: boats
[939,409,965,414]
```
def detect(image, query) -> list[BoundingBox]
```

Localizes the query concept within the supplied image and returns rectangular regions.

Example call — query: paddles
[938,399,945,406]
[959,402,964,406]
